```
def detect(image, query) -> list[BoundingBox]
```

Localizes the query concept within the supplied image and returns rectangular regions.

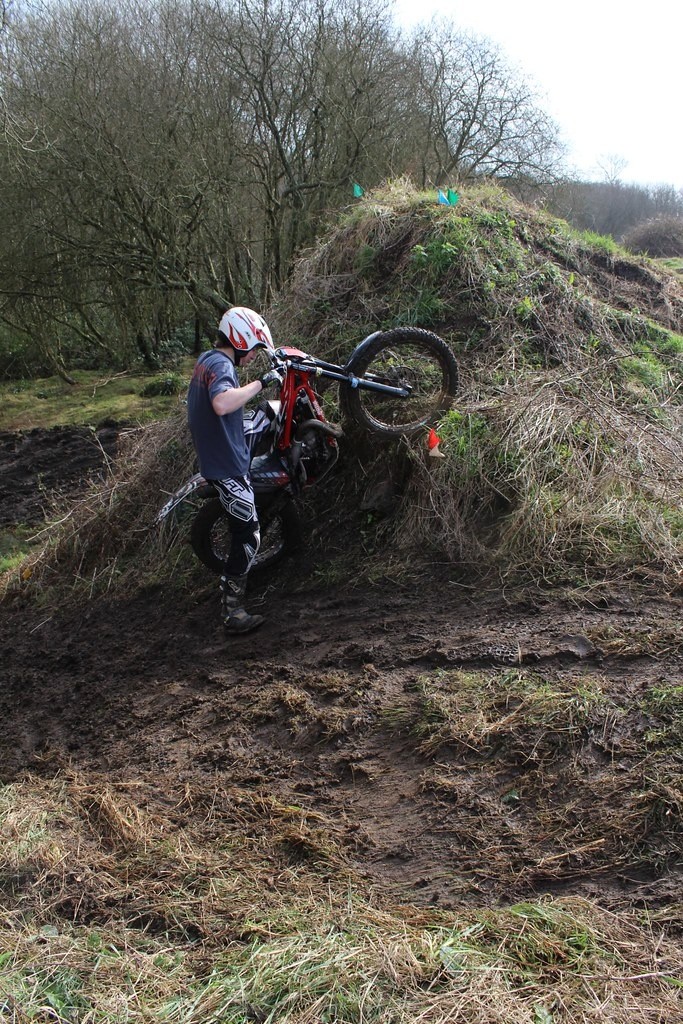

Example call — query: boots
[221,568,265,635]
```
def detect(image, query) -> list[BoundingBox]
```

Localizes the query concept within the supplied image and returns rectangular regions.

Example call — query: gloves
[256,369,284,389]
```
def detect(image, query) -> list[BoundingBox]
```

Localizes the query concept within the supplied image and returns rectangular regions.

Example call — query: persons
[185,304,286,637]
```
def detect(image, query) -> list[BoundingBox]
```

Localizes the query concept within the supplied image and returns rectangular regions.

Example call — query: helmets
[218,306,275,367]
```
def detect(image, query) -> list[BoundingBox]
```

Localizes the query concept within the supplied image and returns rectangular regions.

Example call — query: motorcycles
[155,326,461,574]
